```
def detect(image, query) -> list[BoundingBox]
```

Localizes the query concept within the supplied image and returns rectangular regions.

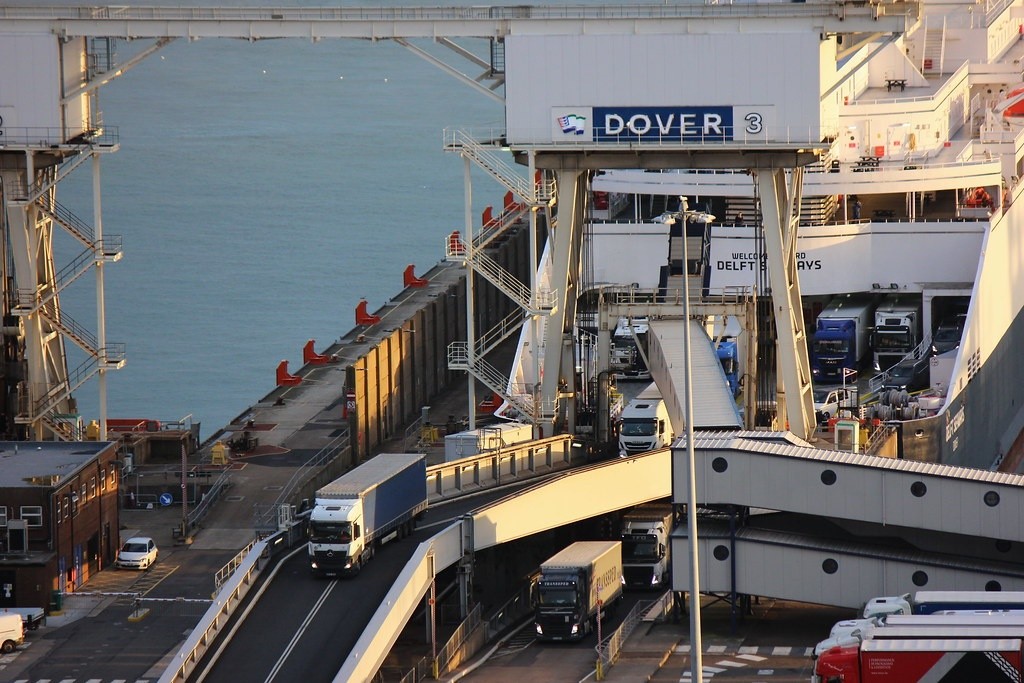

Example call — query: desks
[885,79,906,92]
[850,155,882,172]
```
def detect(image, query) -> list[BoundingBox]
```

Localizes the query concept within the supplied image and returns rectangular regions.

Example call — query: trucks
[702,315,749,402]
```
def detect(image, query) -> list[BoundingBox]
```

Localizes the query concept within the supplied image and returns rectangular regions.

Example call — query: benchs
[873,209,896,217]
[886,83,907,92]
[957,204,992,222]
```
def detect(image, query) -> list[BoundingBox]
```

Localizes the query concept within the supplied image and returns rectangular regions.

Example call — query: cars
[0,612,25,654]
[114,537,159,571]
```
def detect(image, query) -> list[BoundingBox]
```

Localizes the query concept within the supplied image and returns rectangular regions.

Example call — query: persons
[341,527,356,539]
[735,212,744,225]
[851,200,862,224]
[959,191,988,208]
[1002,190,1007,208]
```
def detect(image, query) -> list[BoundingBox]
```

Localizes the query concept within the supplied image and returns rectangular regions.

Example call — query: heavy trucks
[612,316,652,380]
[620,499,674,593]
[527,539,625,643]
[869,294,923,376]
[306,452,429,581]
[618,380,673,458]
[809,292,877,385]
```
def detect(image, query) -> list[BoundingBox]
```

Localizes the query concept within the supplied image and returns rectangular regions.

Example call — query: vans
[812,385,858,420]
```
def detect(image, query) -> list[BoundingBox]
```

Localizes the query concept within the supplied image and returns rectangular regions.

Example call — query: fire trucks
[809,587,1023,683]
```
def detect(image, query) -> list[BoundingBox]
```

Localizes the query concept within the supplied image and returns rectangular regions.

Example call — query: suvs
[931,297,974,358]
[880,358,930,393]
